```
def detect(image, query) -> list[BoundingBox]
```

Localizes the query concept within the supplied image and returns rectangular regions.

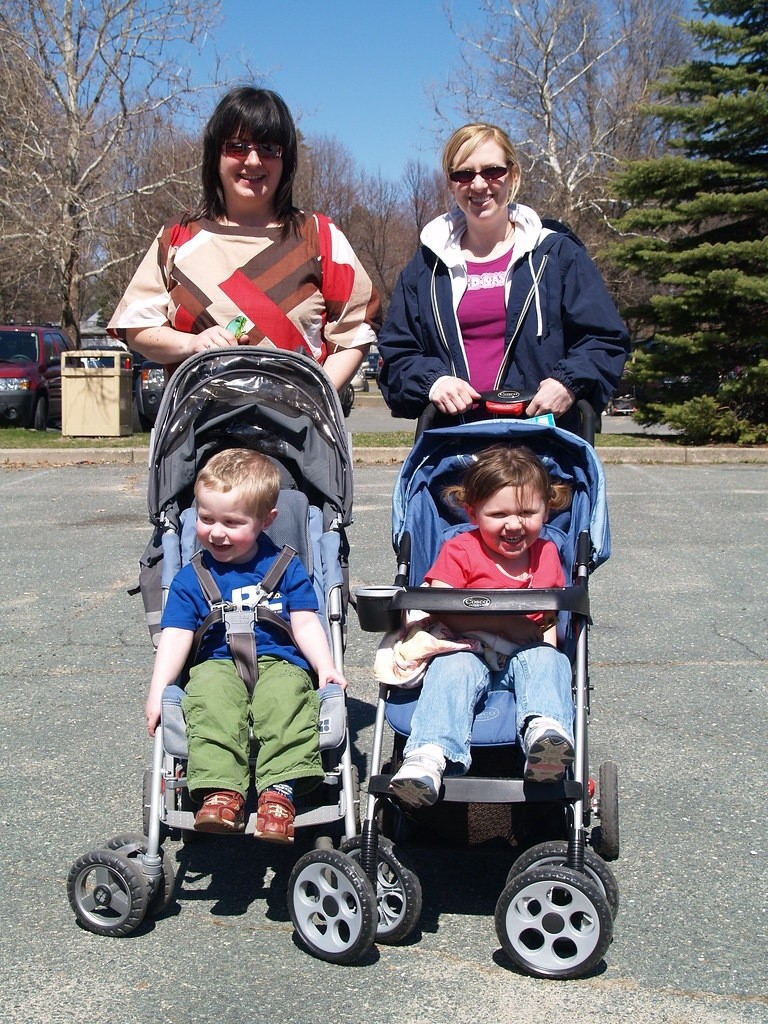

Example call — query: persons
[146,449,349,846]
[378,123,632,858]
[388,442,575,810]
[106,85,383,396]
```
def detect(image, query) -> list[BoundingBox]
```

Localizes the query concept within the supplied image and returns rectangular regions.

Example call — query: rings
[206,341,214,349]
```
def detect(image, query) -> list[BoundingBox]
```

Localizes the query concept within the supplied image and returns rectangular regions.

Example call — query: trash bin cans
[60,350,134,438]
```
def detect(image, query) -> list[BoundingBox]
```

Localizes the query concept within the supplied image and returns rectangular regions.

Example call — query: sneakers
[194,790,245,833]
[253,791,295,844]
[387,754,441,809]
[522,716,576,787]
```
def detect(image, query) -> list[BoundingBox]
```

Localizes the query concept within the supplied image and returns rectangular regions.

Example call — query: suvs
[0,324,79,432]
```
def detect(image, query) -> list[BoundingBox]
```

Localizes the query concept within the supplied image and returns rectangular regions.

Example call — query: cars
[135,362,167,434]
[79,345,131,369]
[339,352,383,416]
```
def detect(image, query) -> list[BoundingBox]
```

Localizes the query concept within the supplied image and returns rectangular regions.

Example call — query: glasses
[449,163,513,184]
[221,141,283,159]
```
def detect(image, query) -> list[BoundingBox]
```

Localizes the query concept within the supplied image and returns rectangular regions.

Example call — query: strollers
[68,345,425,965]
[335,388,620,981]
